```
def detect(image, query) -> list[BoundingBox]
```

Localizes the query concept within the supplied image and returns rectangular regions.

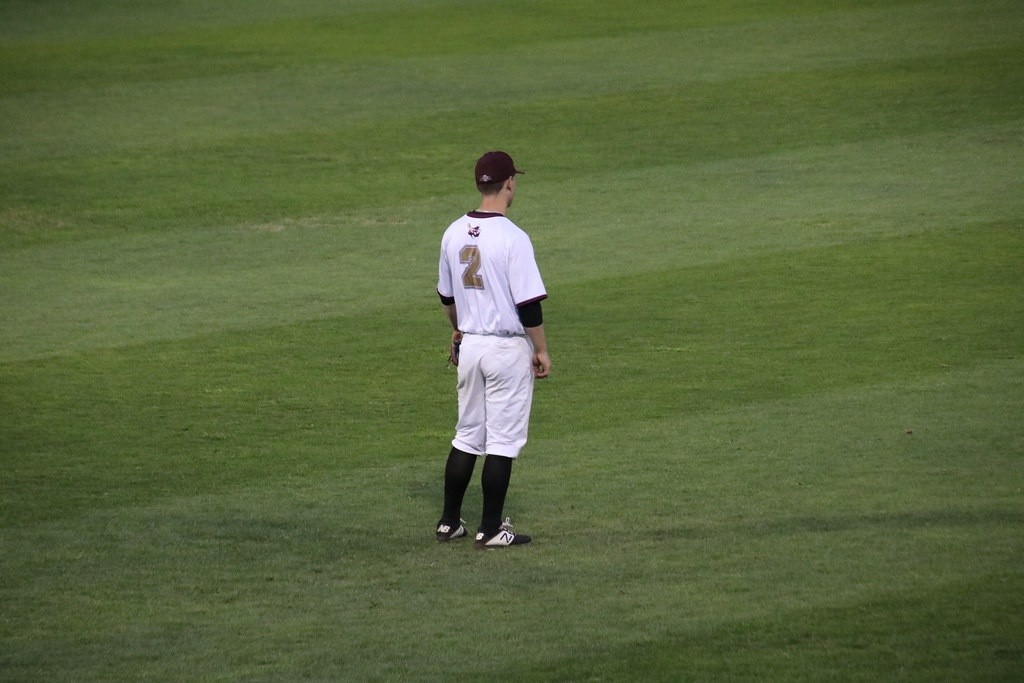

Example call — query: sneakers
[475,516,531,550]
[435,518,469,542]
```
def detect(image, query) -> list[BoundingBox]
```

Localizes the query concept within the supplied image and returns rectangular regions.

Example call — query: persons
[437,151,551,548]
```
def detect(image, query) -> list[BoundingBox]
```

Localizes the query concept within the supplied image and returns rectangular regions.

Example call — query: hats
[475,151,525,184]
